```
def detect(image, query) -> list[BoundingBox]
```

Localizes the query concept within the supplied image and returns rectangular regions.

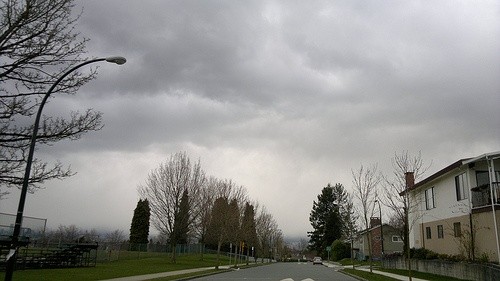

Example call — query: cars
[313,257,322,265]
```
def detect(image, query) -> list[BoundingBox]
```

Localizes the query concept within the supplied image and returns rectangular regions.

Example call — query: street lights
[5,55,127,281]
[375,200,384,254]
